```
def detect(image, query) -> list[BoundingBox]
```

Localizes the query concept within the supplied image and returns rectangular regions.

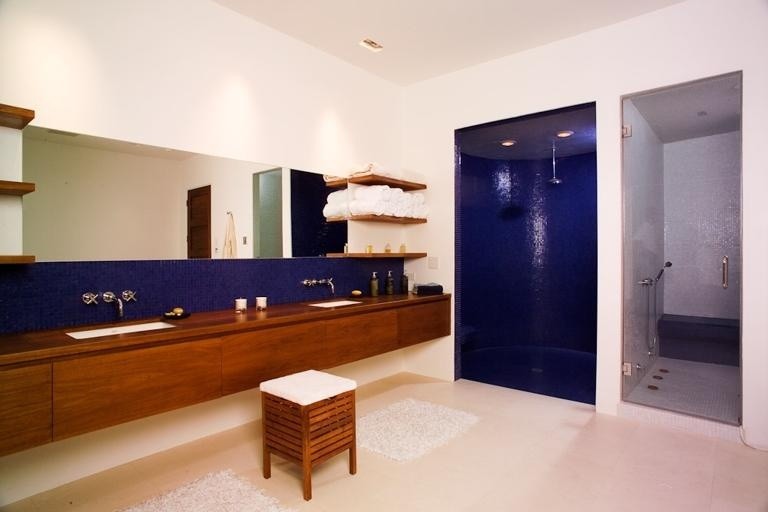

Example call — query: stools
[259,369,356,504]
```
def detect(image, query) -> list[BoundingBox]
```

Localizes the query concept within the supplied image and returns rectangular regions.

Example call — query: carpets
[356,399,480,465]
[112,467,290,512]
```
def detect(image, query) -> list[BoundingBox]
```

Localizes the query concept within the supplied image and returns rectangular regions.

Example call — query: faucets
[103,292,124,316]
[318,278,334,293]
[656,261,672,281]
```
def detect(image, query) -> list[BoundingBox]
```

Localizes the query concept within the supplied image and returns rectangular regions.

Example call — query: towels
[322,183,429,219]
[222,212,237,258]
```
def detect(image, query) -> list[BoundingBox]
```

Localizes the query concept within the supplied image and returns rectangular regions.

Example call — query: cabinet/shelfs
[324,174,432,259]
[0,102,36,265]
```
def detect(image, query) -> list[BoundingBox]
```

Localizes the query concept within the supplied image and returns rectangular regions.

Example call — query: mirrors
[21,120,349,264]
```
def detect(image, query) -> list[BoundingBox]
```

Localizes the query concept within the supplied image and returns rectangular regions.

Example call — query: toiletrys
[344,243,349,253]
[385,270,394,295]
[400,242,407,253]
[370,272,380,297]
[367,239,372,253]
[387,271,393,295]
[372,272,378,297]
[412,283,417,292]
[399,273,408,294]
[384,240,392,252]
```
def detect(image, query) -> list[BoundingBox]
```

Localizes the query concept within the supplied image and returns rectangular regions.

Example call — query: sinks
[307,301,363,308]
[65,321,176,340]
